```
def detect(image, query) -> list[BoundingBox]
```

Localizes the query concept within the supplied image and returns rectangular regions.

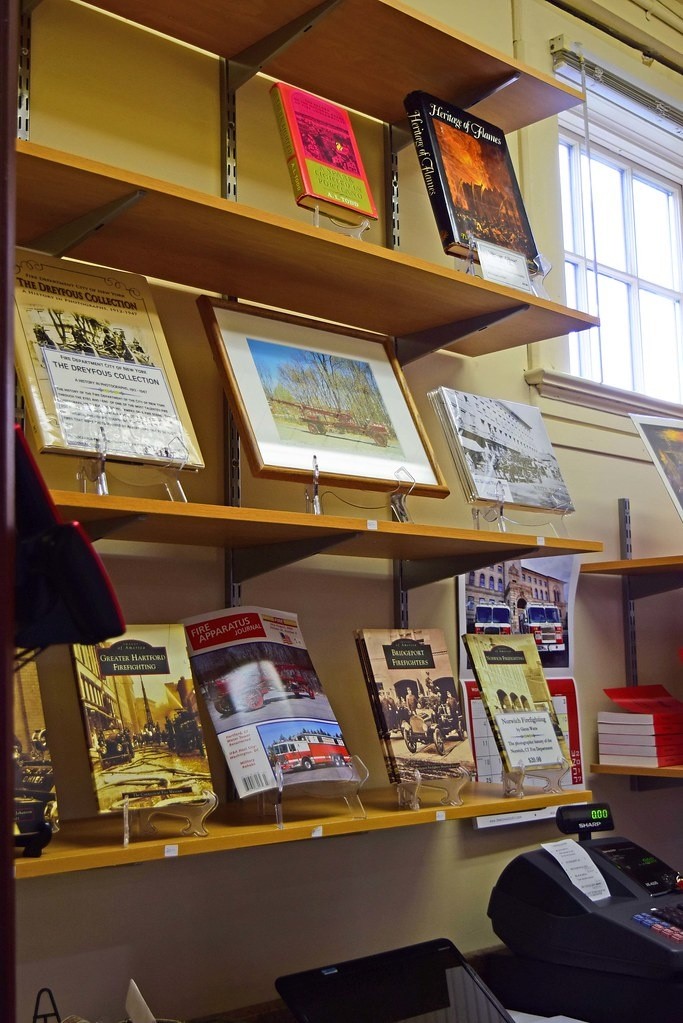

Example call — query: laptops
[276,938,516,1023]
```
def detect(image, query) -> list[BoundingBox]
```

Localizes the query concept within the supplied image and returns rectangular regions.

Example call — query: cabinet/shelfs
[10,0,594,878]
[579,554,683,793]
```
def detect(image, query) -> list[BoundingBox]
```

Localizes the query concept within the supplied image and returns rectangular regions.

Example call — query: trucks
[474,601,512,635]
[518,602,566,656]
[273,734,351,770]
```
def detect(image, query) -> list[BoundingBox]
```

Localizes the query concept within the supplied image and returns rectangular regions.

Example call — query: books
[14,247,207,473]
[71,623,214,814]
[14,646,60,838]
[461,634,574,774]
[353,628,478,785]
[180,606,362,799]
[426,386,576,515]
[270,82,378,230]
[597,684,683,769]
[402,91,545,279]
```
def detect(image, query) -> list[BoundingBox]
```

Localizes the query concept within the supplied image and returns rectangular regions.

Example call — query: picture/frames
[195,294,451,500]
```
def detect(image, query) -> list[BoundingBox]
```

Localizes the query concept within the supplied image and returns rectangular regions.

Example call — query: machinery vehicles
[271,398,390,447]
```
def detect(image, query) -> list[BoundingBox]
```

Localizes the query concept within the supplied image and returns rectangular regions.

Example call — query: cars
[97,728,134,769]
[207,663,315,714]
[400,696,465,755]
